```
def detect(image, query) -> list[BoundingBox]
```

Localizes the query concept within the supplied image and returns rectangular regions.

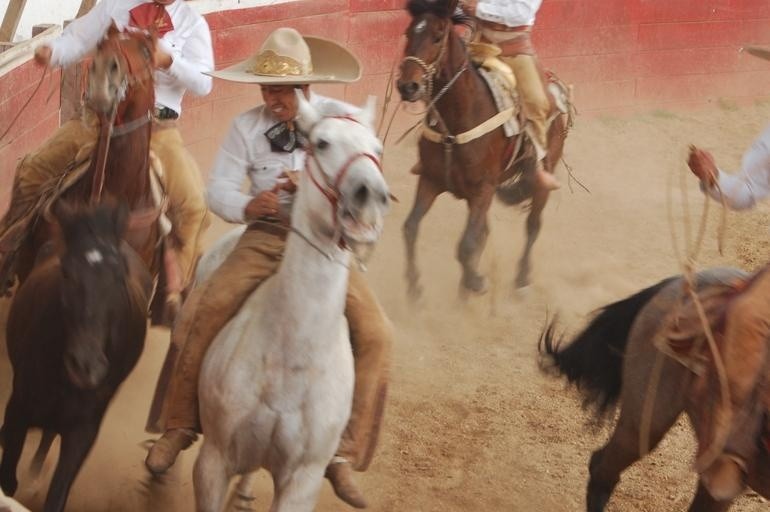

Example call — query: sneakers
[326,457,370,510]
[144,427,198,476]
[526,166,563,191]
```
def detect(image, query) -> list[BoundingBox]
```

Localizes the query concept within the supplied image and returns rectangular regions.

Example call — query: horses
[185,86,392,511]
[536,265,770,511]
[396,0,572,312]
[0,184,148,512]
[18,16,169,332]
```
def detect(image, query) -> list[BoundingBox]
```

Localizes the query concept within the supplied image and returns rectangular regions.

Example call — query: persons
[145,25,398,510]
[685,118,769,217]
[453,0,563,190]
[1,0,217,329]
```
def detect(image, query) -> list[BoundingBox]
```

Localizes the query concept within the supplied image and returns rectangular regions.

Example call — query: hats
[198,25,364,87]
